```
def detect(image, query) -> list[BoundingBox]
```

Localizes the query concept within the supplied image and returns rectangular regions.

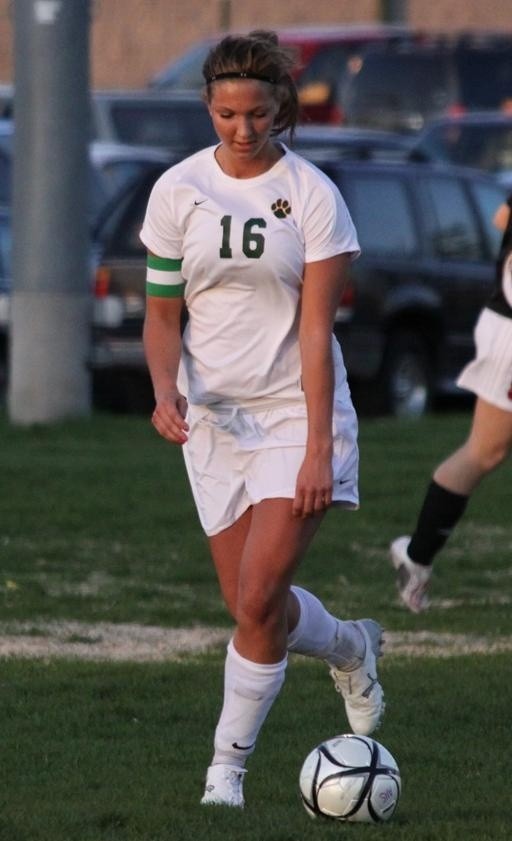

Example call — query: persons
[140,28,385,808]
[390,193,512,617]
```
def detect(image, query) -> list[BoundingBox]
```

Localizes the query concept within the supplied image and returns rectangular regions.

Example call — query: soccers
[299,734,401,822]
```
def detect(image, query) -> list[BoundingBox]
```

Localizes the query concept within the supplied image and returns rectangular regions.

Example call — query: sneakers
[327,617,387,738]
[390,537,432,614]
[201,763,245,808]
